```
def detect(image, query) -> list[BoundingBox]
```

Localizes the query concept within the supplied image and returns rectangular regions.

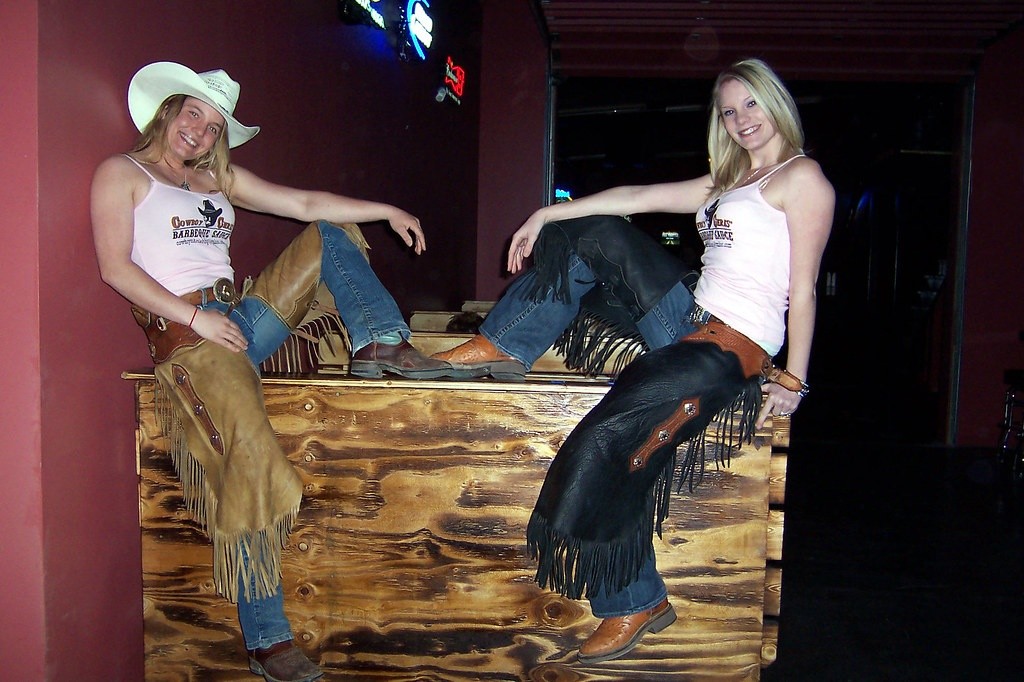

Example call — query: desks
[120,359,774,682]
[318,324,780,669]
[408,306,491,333]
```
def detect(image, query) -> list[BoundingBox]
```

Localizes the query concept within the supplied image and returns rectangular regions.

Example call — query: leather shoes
[429,333,527,382]
[577,598,677,664]
[248,640,326,682]
[350,338,454,379]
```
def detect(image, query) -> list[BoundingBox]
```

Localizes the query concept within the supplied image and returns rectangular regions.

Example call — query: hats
[127,60,261,150]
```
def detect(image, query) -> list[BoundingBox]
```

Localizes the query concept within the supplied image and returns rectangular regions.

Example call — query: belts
[689,302,725,330]
[147,276,237,325]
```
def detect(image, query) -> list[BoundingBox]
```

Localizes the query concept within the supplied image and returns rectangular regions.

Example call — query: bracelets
[767,365,809,398]
[188,306,200,328]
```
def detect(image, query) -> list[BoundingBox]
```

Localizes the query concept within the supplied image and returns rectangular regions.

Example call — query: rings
[780,410,788,417]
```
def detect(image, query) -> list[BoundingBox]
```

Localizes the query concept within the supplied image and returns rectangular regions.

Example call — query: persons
[423,54,837,664]
[86,59,454,682]
